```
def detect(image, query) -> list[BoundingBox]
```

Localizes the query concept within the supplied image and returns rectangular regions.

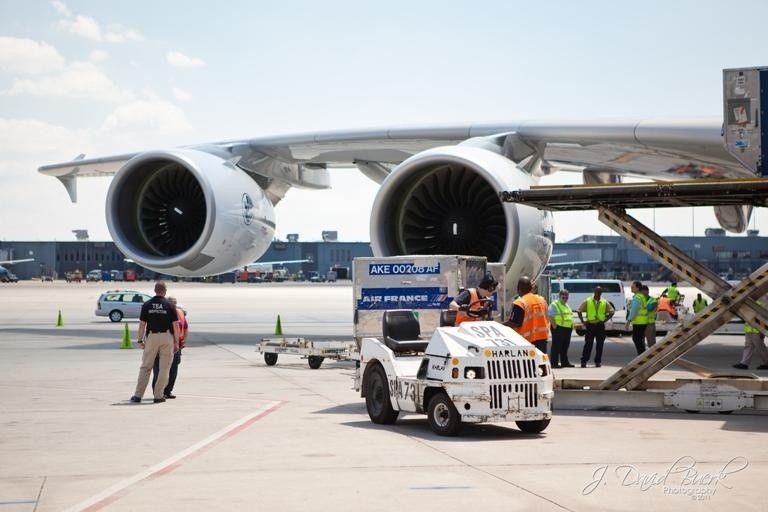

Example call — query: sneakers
[154,398,165,403]
[164,393,176,398]
[131,396,141,402]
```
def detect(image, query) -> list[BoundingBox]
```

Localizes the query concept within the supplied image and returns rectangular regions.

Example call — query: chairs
[382,309,430,357]
[440,309,458,328]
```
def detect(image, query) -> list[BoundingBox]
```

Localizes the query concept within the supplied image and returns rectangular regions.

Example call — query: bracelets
[137,341,143,343]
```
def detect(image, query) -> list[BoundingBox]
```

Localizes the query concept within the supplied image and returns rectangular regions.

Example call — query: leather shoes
[756,365,767,370]
[580,362,586,367]
[596,363,601,367]
[561,363,574,367]
[551,365,562,368]
[732,363,748,369]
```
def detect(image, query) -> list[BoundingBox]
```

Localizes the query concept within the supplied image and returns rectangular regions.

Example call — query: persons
[624,282,681,356]
[576,286,616,368]
[501,275,549,357]
[151,297,188,399]
[732,296,768,370]
[547,289,575,368]
[692,294,708,315]
[131,281,179,403]
[449,273,498,328]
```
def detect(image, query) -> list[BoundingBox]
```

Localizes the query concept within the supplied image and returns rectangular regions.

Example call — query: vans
[549,279,625,317]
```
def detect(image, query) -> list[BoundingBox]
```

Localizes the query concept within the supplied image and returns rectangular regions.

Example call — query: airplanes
[0,257,35,281]
[38,120,768,301]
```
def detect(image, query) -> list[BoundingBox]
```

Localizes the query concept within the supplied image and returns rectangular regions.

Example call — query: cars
[96,290,187,323]
[62,267,342,282]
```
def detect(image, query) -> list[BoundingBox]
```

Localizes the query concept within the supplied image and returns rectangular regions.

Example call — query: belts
[151,331,166,333]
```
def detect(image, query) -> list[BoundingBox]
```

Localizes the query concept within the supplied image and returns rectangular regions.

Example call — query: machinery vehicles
[355,309,556,437]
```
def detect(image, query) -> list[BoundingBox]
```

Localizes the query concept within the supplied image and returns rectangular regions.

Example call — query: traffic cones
[123,322,134,353]
[54,309,63,327]
[272,314,284,335]
